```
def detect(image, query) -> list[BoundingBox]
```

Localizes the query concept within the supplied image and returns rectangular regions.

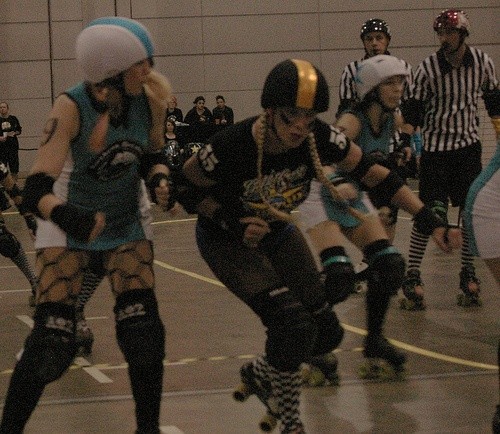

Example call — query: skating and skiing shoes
[298,354,339,388]
[359,335,408,381]
[456,268,484,308]
[73,316,93,356]
[28,279,39,305]
[232,355,281,432]
[401,272,427,311]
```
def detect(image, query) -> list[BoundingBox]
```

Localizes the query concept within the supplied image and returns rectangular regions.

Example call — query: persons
[401,8,500,311]
[0,17,176,434]
[175,58,462,434]
[0,161,94,357]
[163,94,234,172]
[0,102,22,183]
[299,54,412,387]
[337,18,414,293]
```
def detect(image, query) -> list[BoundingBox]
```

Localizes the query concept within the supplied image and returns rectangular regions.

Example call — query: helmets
[77,16,154,84]
[261,59,330,113]
[360,19,390,40]
[433,10,471,37]
[356,56,409,102]
